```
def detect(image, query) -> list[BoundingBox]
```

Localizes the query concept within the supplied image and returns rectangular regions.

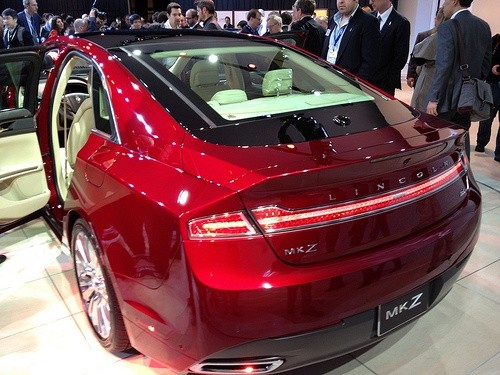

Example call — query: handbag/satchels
[457,75,494,122]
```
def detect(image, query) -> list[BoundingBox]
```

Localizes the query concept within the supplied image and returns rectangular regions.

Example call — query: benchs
[209,67,309,108]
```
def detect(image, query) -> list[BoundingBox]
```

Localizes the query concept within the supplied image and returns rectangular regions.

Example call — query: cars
[0,27,484,375]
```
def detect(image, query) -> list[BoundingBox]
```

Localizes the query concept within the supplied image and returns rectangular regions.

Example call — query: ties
[30,16,36,35]
[378,16,382,22]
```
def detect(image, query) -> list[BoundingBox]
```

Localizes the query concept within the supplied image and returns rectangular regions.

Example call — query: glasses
[267,23,278,29]
[292,6,297,11]
[3,17,12,20]
[256,18,262,23]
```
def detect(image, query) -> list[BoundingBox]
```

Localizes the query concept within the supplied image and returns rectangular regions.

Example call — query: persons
[407,0,500,163]
[237,20,247,29]
[0,0,222,71]
[243,0,410,97]
[224,17,234,28]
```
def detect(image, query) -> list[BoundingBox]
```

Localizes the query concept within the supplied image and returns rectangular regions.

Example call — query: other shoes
[494,154,500,161]
[475,145,485,152]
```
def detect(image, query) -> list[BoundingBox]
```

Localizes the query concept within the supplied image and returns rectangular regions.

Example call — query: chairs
[66,69,95,167]
[188,60,220,102]
